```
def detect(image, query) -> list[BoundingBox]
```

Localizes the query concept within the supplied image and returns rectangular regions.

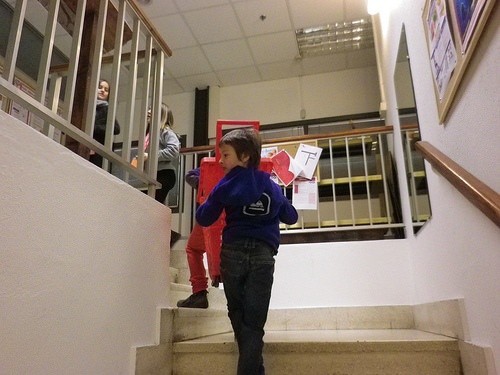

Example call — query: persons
[177,149,227,309]
[195,129,299,375]
[90,79,120,174]
[131,101,181,204]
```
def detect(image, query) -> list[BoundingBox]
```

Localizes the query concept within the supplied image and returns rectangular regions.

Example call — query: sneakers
[177,289,209,309]
[211,277,220,288]
